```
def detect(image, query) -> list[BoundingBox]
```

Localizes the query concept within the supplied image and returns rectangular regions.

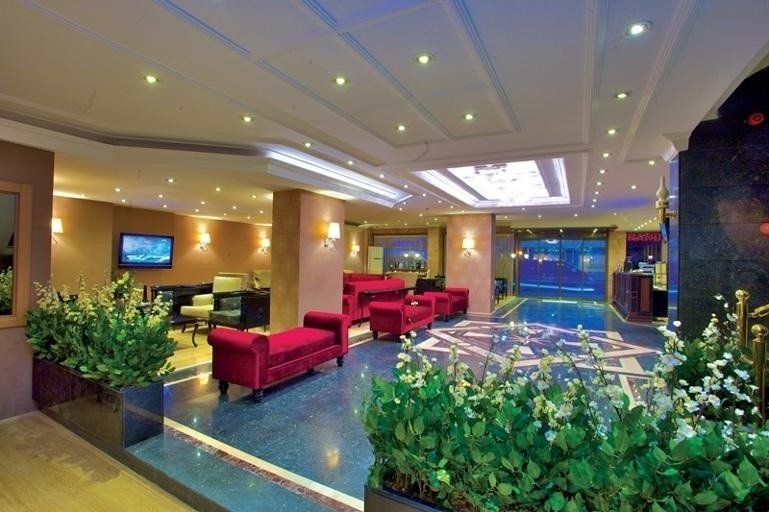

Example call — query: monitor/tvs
[118,232,174,269]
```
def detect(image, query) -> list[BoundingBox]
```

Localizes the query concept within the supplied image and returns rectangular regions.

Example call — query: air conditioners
[368,246,383,274]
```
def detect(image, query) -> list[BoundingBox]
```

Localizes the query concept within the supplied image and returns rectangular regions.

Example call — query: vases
[33,354,163,450]
[363,482,440,512]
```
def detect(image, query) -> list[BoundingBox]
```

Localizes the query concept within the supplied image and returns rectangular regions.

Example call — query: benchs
[208,287,469,403]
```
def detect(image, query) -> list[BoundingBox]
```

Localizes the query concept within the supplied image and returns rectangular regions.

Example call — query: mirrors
[0,181,34,329]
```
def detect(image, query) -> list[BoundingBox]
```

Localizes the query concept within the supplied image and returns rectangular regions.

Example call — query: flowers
[368,296,769,512]
[29,271,177,386]
[0,266,13,307]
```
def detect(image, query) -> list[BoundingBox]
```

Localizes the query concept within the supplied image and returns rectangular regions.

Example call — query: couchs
[343,272,404,327]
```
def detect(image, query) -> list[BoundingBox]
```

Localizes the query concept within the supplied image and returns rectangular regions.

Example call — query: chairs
[151,270,271,348]
